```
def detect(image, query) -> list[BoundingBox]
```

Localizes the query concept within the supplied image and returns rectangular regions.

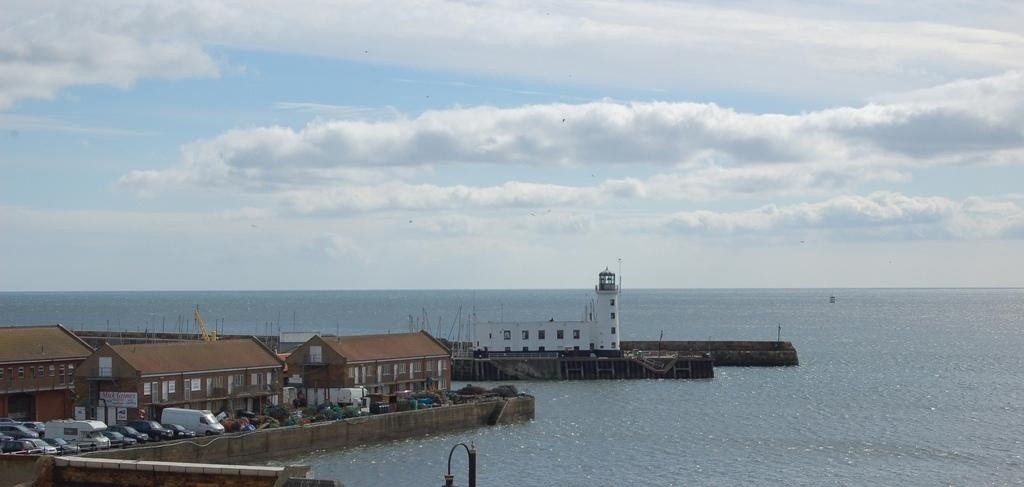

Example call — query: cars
[0,417,81,456]
[103,419,195,448]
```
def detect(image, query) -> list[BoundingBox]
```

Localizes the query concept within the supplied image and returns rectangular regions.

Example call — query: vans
[161,408,225,436]
